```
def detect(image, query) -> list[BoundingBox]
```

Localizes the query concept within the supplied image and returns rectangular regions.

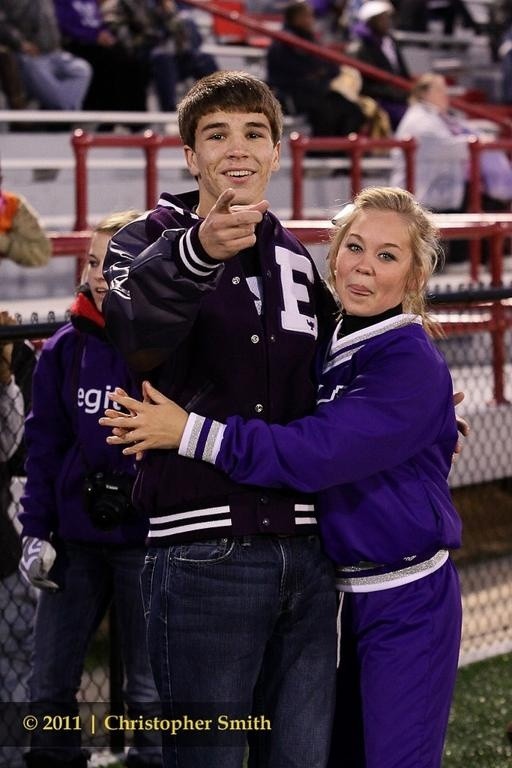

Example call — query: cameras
[80,466,141,533]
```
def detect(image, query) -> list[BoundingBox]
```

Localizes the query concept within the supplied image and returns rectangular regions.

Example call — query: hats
[359,1,393,26]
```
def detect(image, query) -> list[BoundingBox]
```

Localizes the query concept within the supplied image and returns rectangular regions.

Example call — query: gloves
[18,537,60,590]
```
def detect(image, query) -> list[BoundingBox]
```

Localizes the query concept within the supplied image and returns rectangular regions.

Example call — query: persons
[100,186,463,768]
[1,1,510,213]
[16,209,147,766]
[0,173,51,267]
[0,311,40,706]
[101,69,471,768]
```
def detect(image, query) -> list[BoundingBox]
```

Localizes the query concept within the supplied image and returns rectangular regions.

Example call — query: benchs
[2,0,510,245]
